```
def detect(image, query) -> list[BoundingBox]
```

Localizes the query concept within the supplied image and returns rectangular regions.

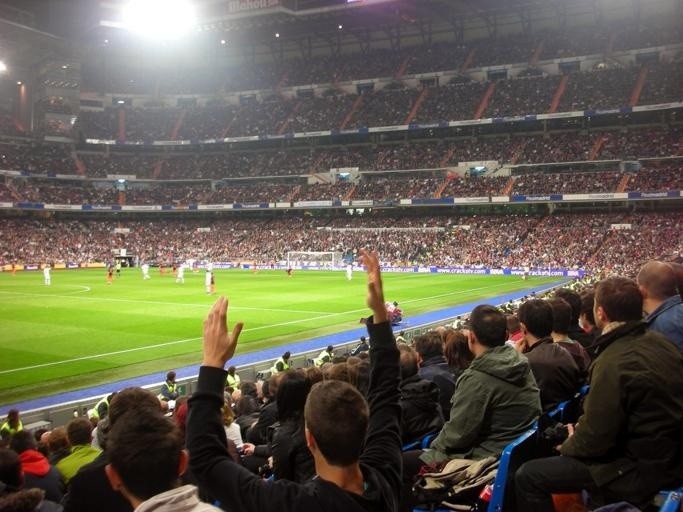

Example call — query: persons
[0,11,683,512]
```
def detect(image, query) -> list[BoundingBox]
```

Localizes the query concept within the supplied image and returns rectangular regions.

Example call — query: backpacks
[413,456,501,511]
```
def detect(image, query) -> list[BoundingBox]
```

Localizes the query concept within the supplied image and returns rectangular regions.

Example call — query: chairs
[214,386,682,512]
